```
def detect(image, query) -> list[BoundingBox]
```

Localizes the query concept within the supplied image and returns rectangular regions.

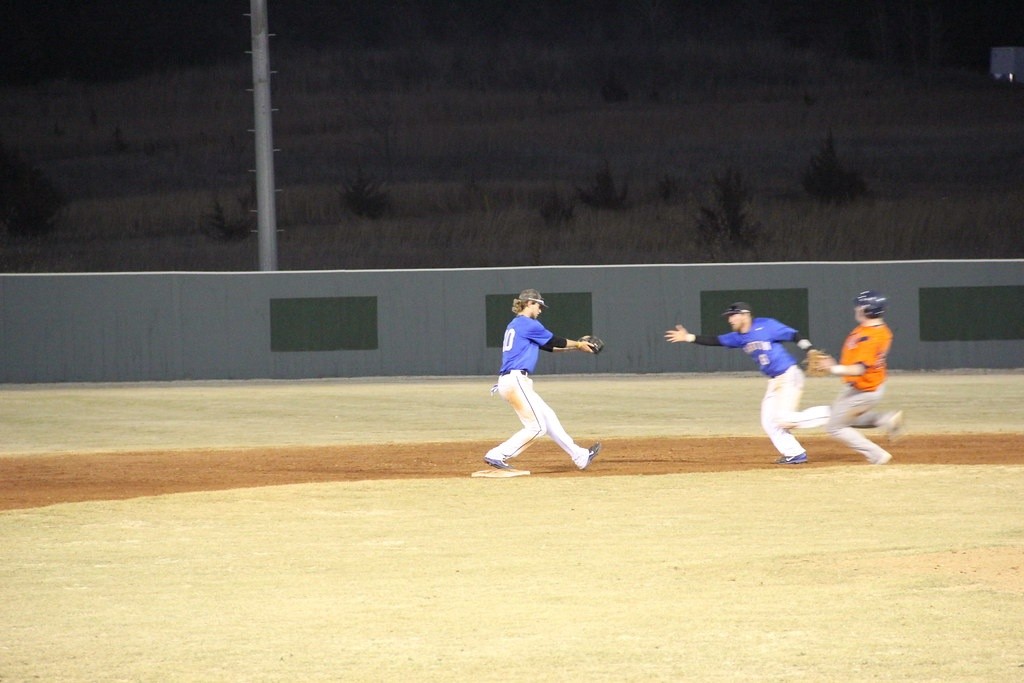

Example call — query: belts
[500,370,526,376]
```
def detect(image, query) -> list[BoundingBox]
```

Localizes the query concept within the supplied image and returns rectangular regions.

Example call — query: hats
[721,302,752,317]
[519,289,550,311]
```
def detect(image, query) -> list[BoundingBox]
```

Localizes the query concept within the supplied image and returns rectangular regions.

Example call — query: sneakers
[770,452,807,465]
[483,457,514,470]
[587,442,601,465]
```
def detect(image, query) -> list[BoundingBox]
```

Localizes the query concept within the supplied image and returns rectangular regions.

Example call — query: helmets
[855,289,886,319]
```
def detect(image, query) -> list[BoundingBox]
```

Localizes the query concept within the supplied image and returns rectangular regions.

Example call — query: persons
[664,302,832,464]
[484,289,603,470]
[817,289,904,464]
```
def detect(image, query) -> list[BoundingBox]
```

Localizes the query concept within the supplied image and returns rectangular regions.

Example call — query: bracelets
[577,342,582,349]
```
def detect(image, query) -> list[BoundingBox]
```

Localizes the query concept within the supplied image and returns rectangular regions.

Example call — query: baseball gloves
[578,335,606,354]
[802,350,832,375]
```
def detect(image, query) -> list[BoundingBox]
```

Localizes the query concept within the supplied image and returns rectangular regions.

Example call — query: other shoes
[886,411,904,435]
[874,452,892,465]
